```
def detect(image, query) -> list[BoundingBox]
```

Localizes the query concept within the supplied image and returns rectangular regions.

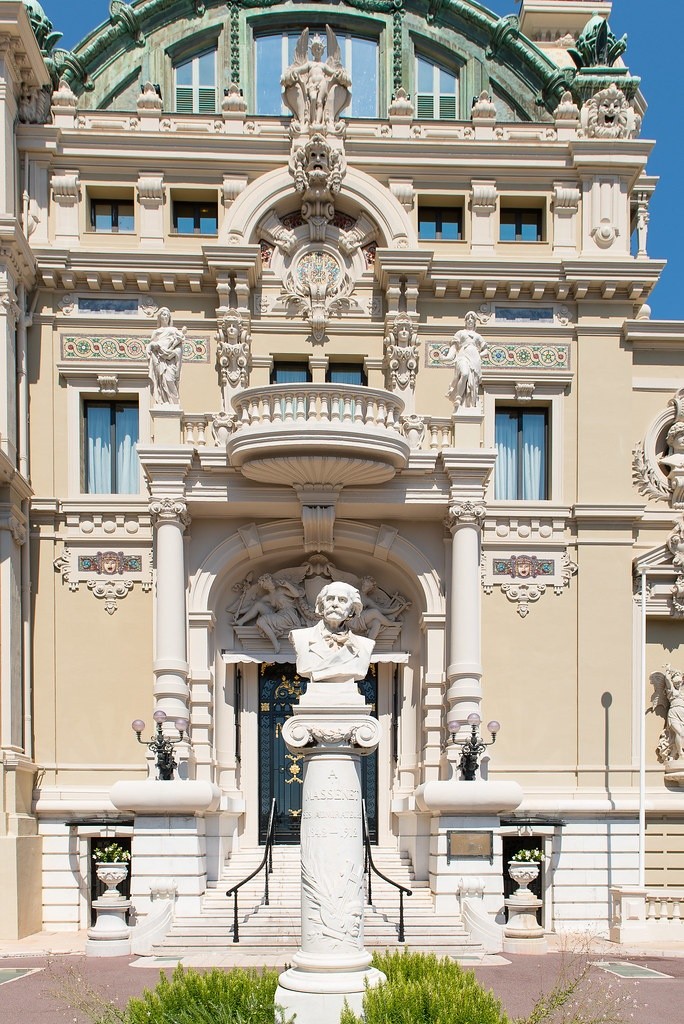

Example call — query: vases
[507,860,541,892]
[95,863,129,894]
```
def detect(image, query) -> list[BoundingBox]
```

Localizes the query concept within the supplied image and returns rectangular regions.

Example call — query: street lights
[448,714,500,781]
[132,710,188,782]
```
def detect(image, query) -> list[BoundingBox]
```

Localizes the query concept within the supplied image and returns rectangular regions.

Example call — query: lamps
[447,713,500,781]
[132,711,190,780]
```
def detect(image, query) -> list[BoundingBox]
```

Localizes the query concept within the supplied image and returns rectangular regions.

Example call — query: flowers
[512,848,545,862]
[92,842,131,863]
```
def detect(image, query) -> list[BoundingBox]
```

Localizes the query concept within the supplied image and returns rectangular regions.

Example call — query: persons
[292,43,339,125]
[662,662,684,761]
[229,573,299,654]
[166,326,186,350]
[147,307,182,405]
[439,311,489,408]
[288,580,376,681]
[346,576,403,640]
